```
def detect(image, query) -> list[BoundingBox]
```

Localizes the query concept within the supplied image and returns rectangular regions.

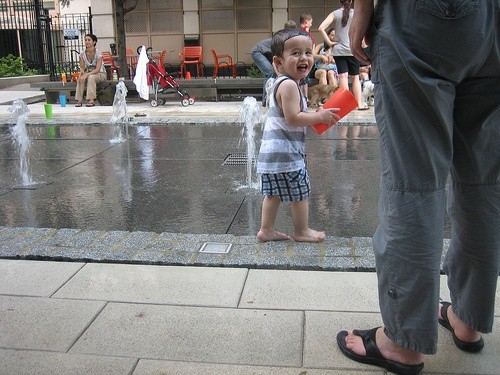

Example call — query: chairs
[126,48,137,67]
[153,49,166,69]
[101,52,120,80]
[211,48,236,78]
[180,46,203,79]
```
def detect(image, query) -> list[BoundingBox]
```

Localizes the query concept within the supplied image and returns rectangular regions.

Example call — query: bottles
[113,70,117,81]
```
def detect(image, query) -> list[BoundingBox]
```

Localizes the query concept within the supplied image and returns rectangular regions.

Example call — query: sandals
[75,102,82,106]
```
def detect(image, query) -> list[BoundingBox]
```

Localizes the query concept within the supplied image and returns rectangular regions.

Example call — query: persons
[318,0,370,110]
[336,0,500,375]
[75,34,107,107]
[284,12,376,110]
[256,29,340,242]
[251,37,274,107]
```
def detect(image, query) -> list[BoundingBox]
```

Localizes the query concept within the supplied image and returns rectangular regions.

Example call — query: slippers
[439,301,485,353]
[337,325,425,375]
[358,105,369,110]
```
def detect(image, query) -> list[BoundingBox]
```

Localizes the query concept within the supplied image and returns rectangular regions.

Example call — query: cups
[59,95,66,107]
[312,86,358,134]
[45,104,52,119]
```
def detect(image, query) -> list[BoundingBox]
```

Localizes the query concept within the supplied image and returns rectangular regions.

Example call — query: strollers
[136,45,196,107]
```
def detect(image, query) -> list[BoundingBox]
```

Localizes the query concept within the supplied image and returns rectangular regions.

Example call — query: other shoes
[86,102,95,106]
